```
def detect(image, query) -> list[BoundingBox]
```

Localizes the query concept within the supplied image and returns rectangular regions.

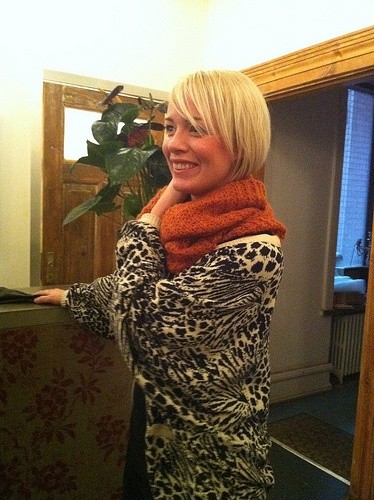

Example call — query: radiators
[331,314,365,384]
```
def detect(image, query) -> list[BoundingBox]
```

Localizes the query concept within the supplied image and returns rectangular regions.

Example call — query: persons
[31,70,288,500]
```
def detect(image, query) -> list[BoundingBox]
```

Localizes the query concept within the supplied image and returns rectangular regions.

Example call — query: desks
[0,300,135,500]
